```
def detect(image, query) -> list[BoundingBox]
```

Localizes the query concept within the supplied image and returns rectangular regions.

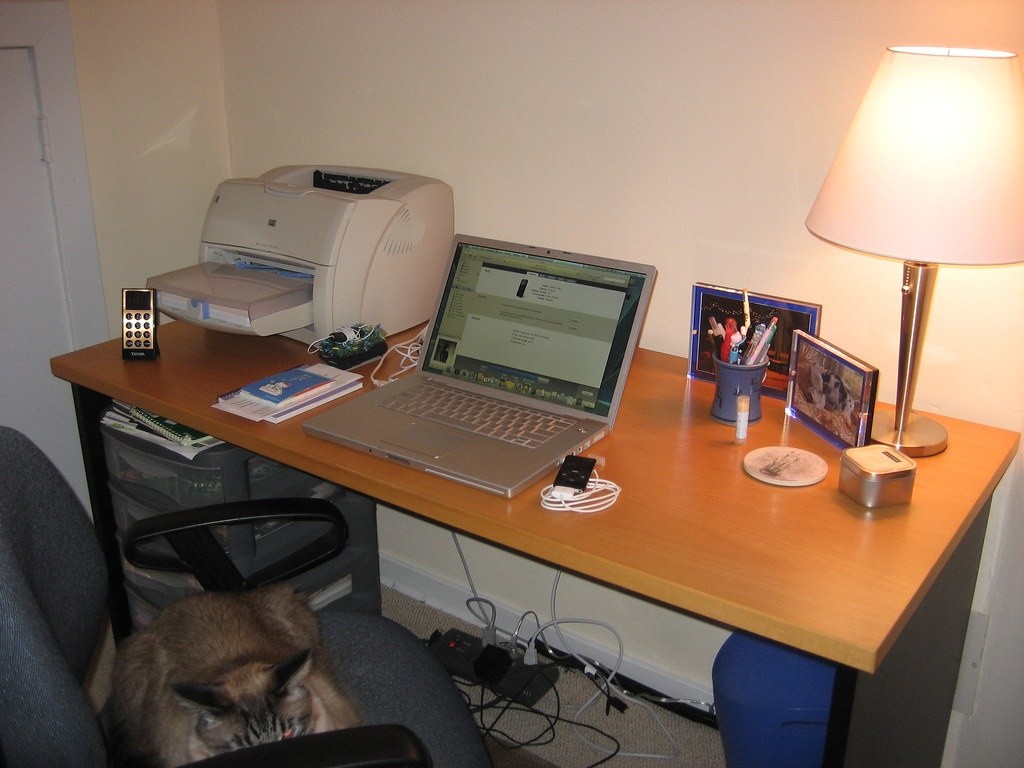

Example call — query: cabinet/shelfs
[100,422,382,631]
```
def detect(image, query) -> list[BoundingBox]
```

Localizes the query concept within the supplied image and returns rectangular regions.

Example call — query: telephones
[121,288,160,362]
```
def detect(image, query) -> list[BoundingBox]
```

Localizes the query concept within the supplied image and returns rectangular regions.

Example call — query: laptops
[301,232,659,499]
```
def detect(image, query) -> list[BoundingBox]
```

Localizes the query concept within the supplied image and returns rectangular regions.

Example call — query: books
[101,363,364,459]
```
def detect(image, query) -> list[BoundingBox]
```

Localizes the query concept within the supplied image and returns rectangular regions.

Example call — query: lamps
[806,46,1024,457]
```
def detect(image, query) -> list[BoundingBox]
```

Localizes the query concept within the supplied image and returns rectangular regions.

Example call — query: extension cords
[427,628,559,706]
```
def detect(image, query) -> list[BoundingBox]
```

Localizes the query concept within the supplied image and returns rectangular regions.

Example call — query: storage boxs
[837,443,917,509]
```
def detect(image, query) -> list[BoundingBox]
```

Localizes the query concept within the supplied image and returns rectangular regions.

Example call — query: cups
[710,351,769,426]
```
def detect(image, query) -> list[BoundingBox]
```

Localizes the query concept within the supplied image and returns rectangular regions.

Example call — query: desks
[51,319,1021,768]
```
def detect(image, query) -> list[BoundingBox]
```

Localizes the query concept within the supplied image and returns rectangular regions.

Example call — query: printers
[145,164,455,350]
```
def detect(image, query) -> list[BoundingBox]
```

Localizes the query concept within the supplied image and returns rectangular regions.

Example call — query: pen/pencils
[707,289,778,365]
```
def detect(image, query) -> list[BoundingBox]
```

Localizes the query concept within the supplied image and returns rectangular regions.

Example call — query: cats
[104,583,362,766]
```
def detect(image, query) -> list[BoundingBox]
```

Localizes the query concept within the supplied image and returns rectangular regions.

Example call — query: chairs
[0,421,500,768]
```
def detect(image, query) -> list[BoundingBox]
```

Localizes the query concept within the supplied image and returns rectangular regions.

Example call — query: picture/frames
[785,328,879,452]
[687,283,822,401]
